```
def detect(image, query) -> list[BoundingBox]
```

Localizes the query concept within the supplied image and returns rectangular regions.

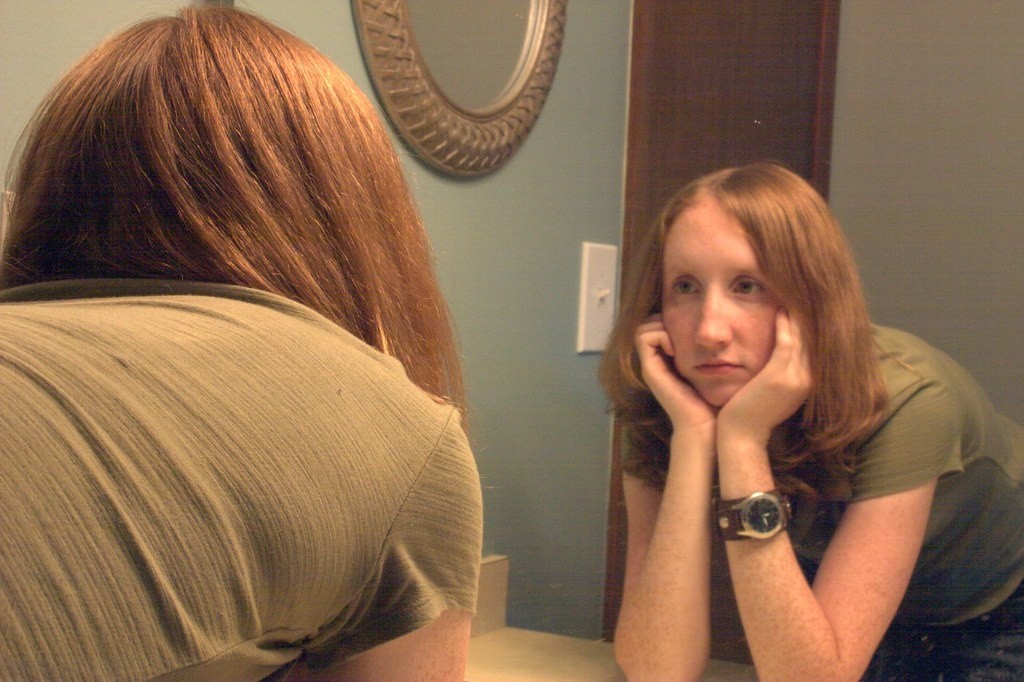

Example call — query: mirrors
[344,0,572,181]
[194,0,1024,682]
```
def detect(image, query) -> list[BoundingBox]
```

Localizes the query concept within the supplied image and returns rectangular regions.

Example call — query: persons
[598,164,1024,682]
[0,6,484,682]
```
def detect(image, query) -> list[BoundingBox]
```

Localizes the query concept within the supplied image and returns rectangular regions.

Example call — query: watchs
[711,491,791,539]
